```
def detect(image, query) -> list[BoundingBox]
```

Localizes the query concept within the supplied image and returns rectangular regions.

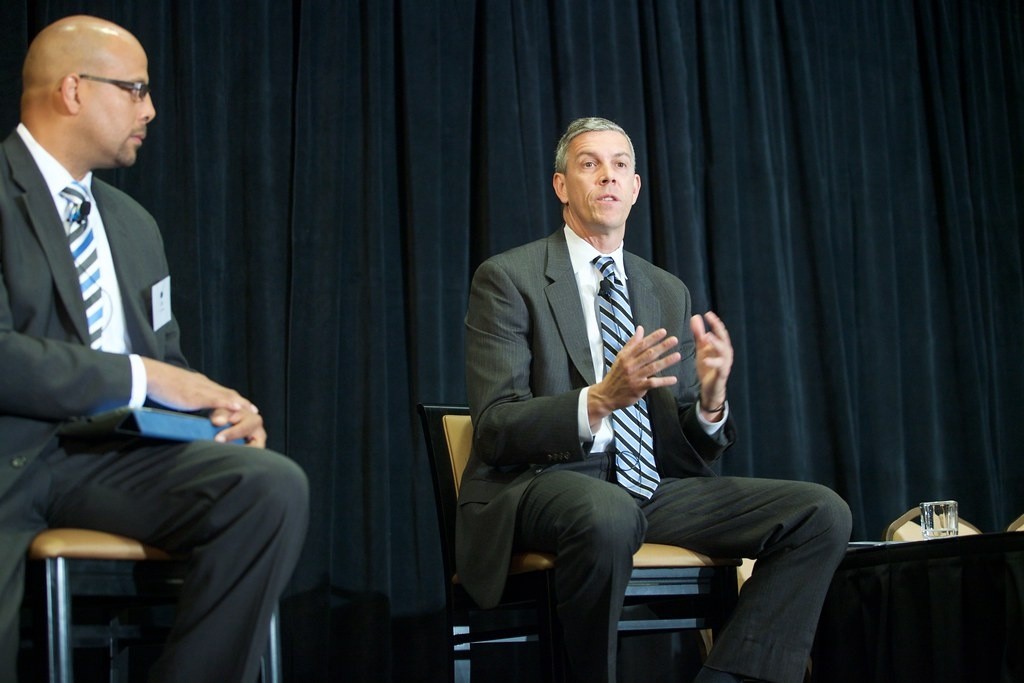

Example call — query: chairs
[420,407,744,682]
[27,529,282,683]
[886,506,982,543]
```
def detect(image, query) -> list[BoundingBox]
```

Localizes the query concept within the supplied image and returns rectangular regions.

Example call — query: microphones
[601,280,611,295]
[80,202,89,217]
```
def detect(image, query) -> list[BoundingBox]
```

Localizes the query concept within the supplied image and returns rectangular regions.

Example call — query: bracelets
[700,394,725,413]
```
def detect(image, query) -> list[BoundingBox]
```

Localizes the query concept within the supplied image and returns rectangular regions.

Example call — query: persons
[0,15,309,683]
[453,117,852,683]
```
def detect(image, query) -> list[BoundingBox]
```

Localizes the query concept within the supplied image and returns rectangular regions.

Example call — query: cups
[920,501,959,541]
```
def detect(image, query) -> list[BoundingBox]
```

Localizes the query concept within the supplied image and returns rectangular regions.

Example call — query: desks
[813,531,1024,683]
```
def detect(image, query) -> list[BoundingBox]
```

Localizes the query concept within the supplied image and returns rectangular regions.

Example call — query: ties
[58,178,104,352]
[592,255,661,500]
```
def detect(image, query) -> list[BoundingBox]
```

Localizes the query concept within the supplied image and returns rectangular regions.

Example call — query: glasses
[58,74,151,101]
